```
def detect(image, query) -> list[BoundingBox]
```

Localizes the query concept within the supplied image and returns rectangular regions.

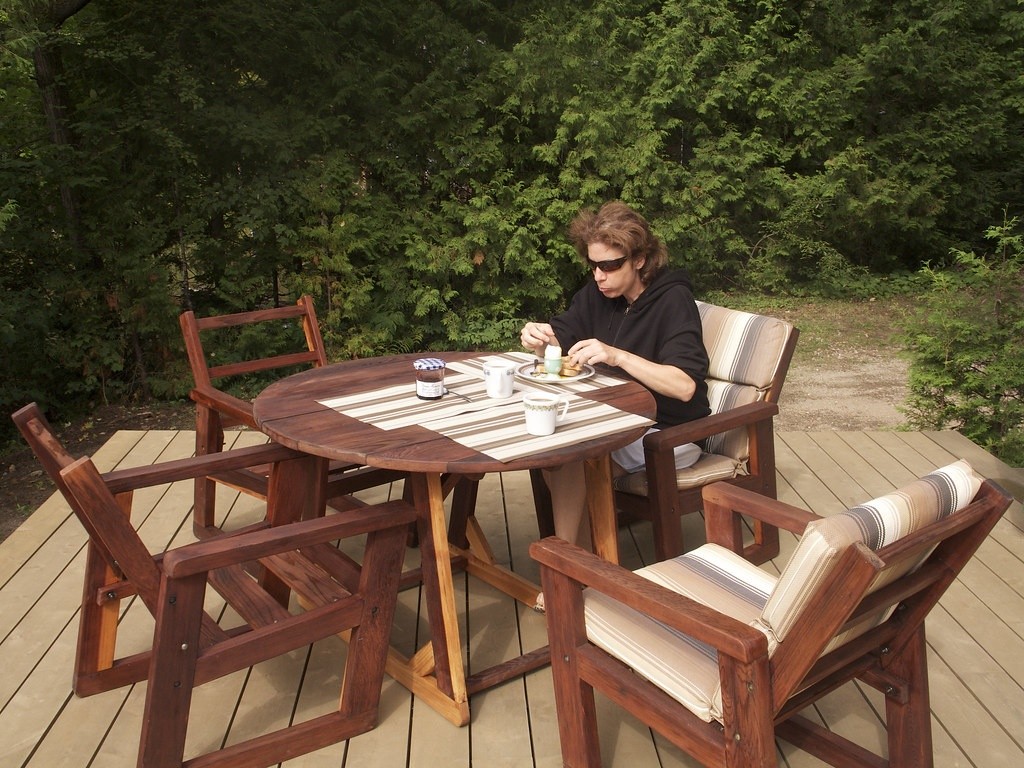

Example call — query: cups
[544,357,562,379]
[483,362,514,398]
[523,393,569,436]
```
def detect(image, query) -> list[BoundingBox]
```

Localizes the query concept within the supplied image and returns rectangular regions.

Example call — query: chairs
[528,459,1017,768]
[529,301,800,567]
[181,296,459,579]
[14,402,416,768]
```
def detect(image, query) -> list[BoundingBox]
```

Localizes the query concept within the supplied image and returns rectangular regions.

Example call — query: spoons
[531,359,539,377]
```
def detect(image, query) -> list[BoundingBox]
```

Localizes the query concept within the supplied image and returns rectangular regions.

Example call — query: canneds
[413,358,446,400]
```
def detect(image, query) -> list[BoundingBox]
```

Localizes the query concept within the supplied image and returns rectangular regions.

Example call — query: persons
[521,201,712,613]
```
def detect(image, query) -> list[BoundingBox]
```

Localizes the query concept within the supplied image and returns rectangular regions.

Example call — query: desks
[252,353,658,729]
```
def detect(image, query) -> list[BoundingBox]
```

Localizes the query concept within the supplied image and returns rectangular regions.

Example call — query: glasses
[585,256,628,272]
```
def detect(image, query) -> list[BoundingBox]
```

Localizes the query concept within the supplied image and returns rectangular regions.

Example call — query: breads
[538,362,581,376]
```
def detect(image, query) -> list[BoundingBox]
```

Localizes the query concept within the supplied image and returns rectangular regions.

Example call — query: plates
[516,361,596,383]
